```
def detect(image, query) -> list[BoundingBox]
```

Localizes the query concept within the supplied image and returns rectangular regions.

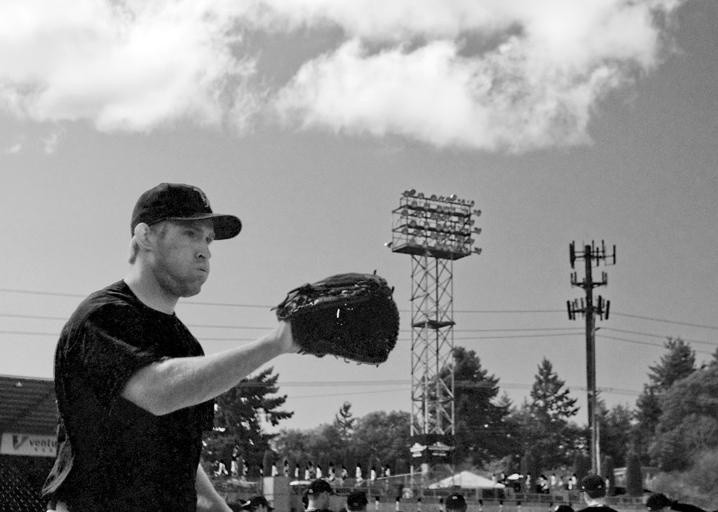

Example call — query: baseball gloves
[272,270,399,368]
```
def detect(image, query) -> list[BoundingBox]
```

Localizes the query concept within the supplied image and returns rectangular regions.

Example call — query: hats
[346,490,367,509]
[445,494,466,509]
[645,492,677,511]
[131,182,242,240]
[301,478,340,505]
[579,473,606,492]
[226,495,276,511]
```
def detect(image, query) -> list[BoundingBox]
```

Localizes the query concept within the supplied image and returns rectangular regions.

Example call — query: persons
[42,182,400,512]
